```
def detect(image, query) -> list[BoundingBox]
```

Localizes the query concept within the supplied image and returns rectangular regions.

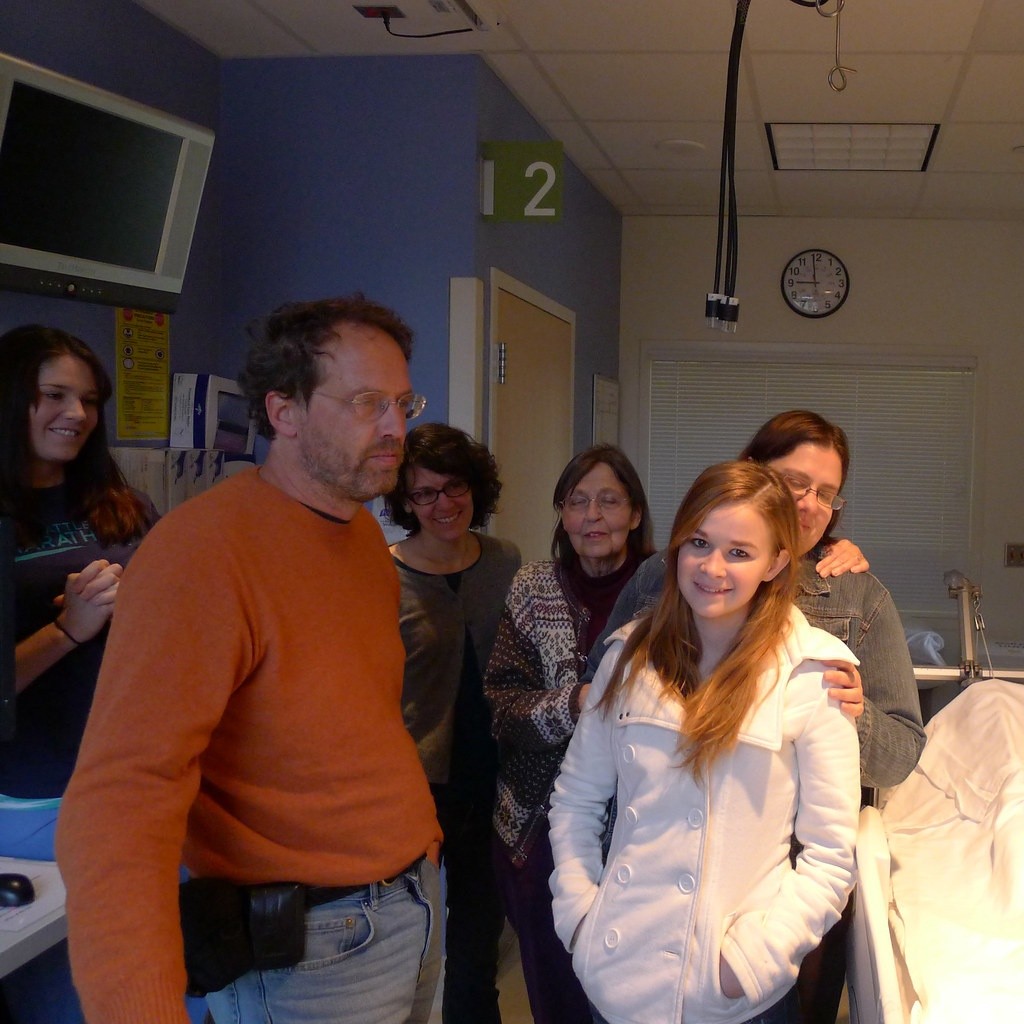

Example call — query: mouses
[0,873,35,908]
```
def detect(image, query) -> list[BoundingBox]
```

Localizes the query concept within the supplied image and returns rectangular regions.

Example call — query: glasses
[558,494,632,509]
[406,477,472,506]
[781,474,846,511]
[314,389,428,420]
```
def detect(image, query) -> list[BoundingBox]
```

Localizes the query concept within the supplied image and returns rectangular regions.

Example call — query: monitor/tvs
[0,53,214,314]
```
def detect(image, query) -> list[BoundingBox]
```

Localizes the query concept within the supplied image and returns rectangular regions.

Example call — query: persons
[53,286,447,1024]
[587,409,924,1024]
[0,324,161,798]
[483,442,870,1024]
[381,418,520,1023]
[550,459,861,1024]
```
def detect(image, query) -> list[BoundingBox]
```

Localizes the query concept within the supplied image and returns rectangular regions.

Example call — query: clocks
[780,249,851,317]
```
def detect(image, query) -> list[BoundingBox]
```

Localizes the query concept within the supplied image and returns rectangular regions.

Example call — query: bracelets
[54,621,82,645]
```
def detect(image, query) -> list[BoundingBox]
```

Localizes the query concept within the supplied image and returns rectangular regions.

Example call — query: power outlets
[353,4,406,18]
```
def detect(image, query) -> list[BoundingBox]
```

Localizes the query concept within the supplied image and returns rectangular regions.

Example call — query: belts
[296,852,428,908]
